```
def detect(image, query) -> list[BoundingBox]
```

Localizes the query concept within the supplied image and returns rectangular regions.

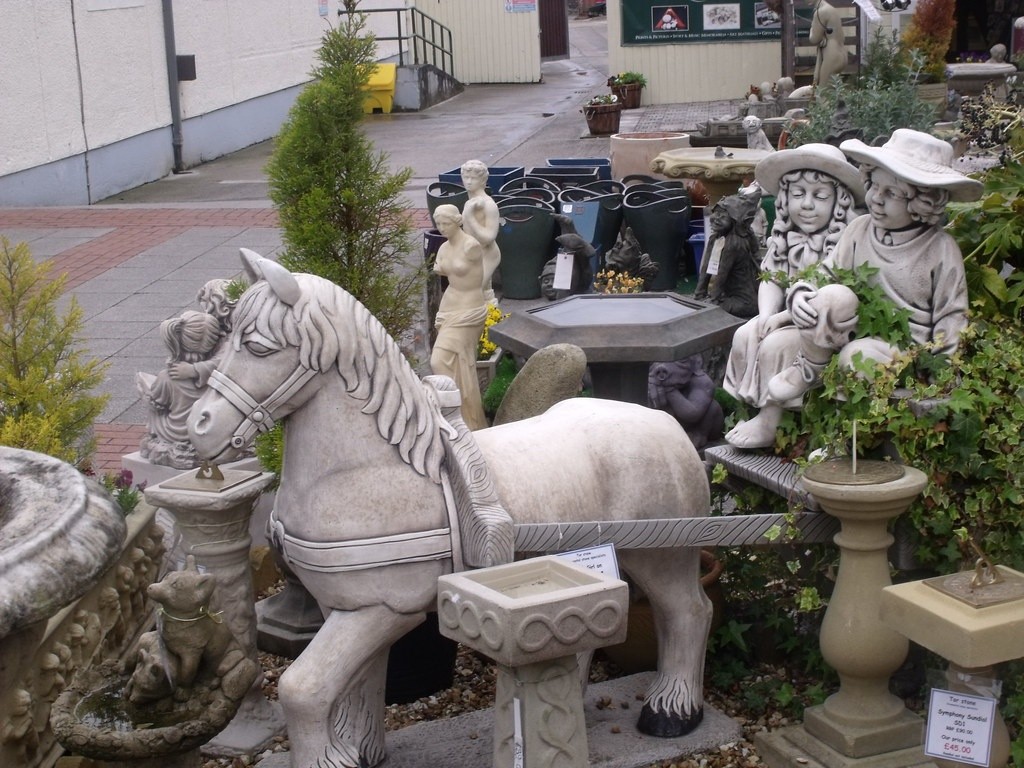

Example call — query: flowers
[606,70,647,88]
[588,93,619,104]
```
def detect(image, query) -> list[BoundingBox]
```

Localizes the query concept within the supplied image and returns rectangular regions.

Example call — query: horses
[186,246,715,767]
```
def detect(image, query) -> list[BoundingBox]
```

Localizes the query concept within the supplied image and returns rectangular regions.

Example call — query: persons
[427,204,488,431]
[695,188,762,314]
[986,44,1012,99]
[459,160,501,311]
[136,278,244,470]
[722,129,984,449]
[537,260,571,300]
[807,0,848,97]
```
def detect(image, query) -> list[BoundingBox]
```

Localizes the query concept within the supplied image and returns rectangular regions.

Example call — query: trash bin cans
[423,228,448,352]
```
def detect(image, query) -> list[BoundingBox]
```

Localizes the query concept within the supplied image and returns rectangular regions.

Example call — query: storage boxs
[422,155,776,303]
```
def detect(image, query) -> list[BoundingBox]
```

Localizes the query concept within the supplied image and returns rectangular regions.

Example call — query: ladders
[782,1,861,90]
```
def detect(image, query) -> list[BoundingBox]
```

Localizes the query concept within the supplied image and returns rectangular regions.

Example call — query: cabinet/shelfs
[782,1,861,91]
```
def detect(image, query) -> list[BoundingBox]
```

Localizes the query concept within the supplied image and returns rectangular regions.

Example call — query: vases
[583,102,622,134]
[611,84,642,108]
